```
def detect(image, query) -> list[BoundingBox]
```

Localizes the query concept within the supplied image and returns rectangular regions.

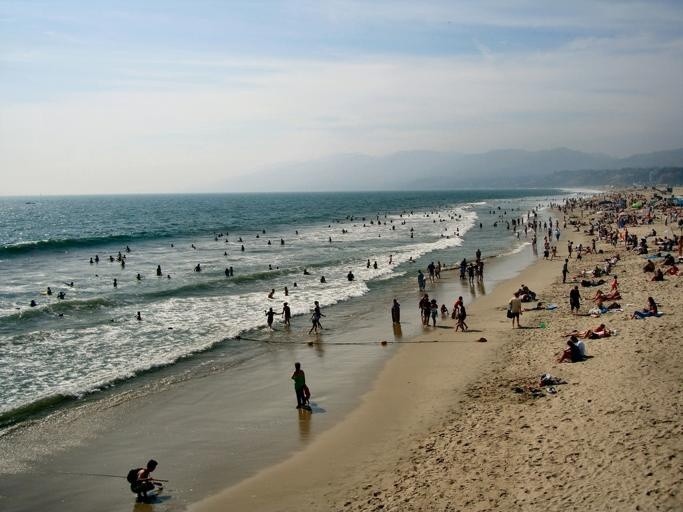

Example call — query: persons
[32,227,333,334]
[559,335,586,362]
[292,363,310,408]
[328,194,683,338]
[132,460,158,498]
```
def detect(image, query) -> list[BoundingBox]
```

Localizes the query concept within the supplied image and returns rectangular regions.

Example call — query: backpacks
[127,468,143,483]
